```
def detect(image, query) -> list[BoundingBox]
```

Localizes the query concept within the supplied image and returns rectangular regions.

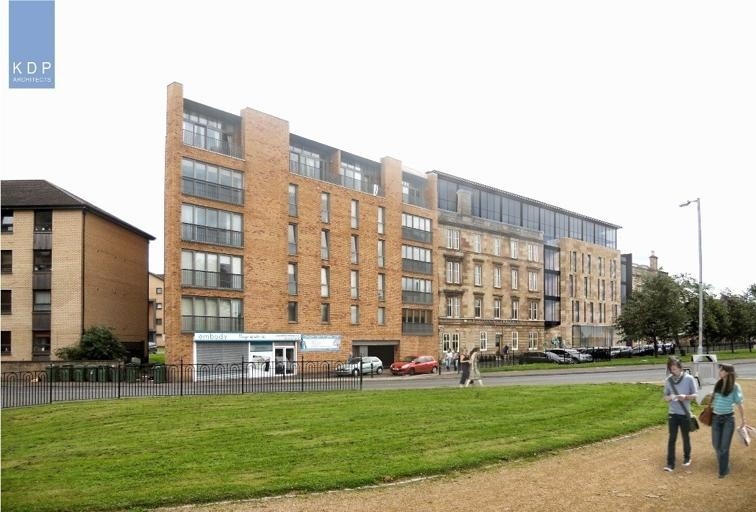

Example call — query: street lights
[679,198,703,355]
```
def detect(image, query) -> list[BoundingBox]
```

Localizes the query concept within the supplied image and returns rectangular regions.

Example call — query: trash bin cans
[152,365,166,384]
[46,363,139,383]
[691,354,718,379]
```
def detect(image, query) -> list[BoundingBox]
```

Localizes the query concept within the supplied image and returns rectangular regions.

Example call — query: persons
[445,347,484,387]
[708,363,746,479]
[663,355,698,471]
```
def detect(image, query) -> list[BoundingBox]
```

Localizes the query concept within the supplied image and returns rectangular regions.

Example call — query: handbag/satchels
[699,407,712,427]
[685,411,699,431]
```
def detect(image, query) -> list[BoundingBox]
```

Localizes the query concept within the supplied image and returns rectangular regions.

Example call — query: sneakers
[682,458,692,466]
[664,464,673,472]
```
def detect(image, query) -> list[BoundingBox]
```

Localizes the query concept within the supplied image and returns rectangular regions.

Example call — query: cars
[335,356,383,376]
[390,354,437,376]
[518,343,673,364]
